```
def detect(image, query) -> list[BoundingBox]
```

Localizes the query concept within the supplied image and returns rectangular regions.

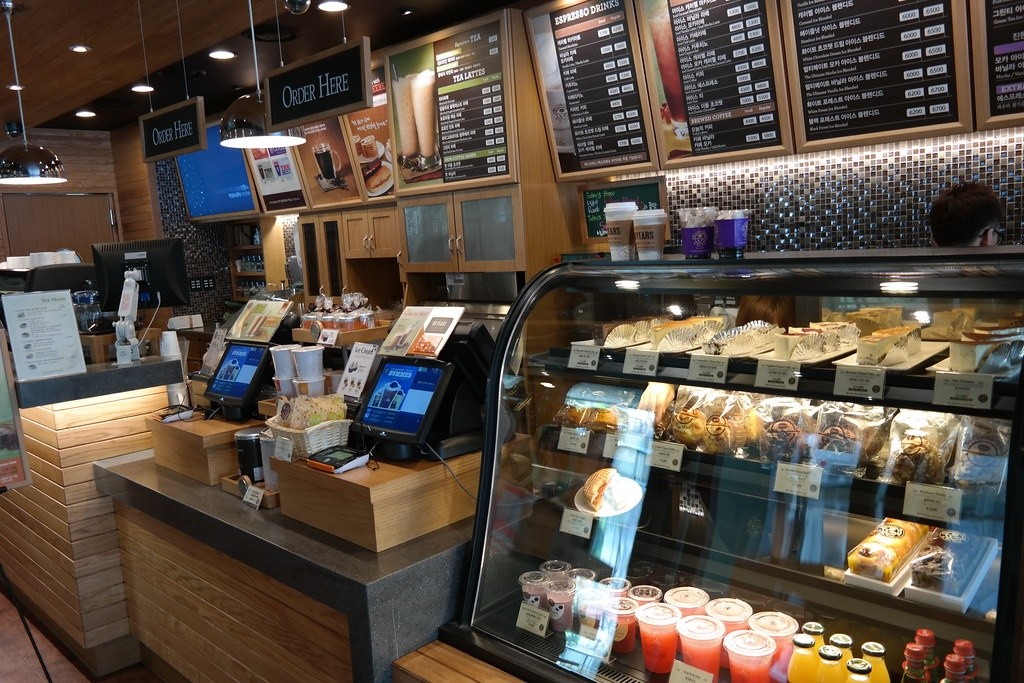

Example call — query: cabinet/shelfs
[344,206,399,259]
[295,212,376,306]
[176,333,211,376]
[225,218,289,301]
[436,246,1024,683]
[398,183,579,273]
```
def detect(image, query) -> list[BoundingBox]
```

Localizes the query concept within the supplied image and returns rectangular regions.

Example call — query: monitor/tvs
[351,356,455,444]
[91,236,192,312]
[204,340,275,407]
[174,119,260,222]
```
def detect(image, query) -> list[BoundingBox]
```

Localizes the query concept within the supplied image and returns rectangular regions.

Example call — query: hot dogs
[365,165,392,192]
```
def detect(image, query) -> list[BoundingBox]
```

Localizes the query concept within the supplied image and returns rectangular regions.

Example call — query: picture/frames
[286,117,366,212]
[244,129,312,217]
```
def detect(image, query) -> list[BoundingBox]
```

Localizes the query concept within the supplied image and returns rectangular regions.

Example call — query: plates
[360,160,394,196]
[358,140,385,163]
[385,143,393,164]
[574,476,642,518]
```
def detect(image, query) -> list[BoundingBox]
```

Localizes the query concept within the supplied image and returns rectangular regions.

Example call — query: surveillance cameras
[5,121,23,138]
[285,0,311,14]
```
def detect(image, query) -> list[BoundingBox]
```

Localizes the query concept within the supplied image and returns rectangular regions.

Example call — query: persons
[731,292,799,330]
[926,181,1007,250]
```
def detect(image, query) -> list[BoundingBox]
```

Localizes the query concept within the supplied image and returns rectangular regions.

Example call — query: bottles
[784,620,979,683]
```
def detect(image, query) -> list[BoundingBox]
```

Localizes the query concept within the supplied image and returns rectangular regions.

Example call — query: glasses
[978,228,1004,246]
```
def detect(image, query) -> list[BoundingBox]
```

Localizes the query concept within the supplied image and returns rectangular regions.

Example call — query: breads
[552,404,947,485]
[849,518,987,598]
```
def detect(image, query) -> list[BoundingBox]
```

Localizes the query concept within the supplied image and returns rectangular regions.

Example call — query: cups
[631,209,667,261]
[644,8,692,140]
[372,380,406,411]
[312,144,343,183]
[269,344,354,417]
[244,313,269,337]
[253,144,283,180]
[674,205,719,261]
[544,88,572,131]
[160,331,190,410]
[219,359,241,381]
[603,202,641,261]
[517,561,801,682]
[409,76,443,171]
[714,209,749,261]
[392,75,423,169]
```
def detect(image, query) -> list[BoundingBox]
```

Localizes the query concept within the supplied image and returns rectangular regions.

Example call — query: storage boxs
[292,320,392,347]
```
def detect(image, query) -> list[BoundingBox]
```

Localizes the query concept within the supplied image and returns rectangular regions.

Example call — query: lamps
[0,0,68,185]
[218,0,307,149]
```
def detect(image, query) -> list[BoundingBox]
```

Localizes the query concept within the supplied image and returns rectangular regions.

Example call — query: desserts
[582,468,619,510]
[588,314,1024,377]
